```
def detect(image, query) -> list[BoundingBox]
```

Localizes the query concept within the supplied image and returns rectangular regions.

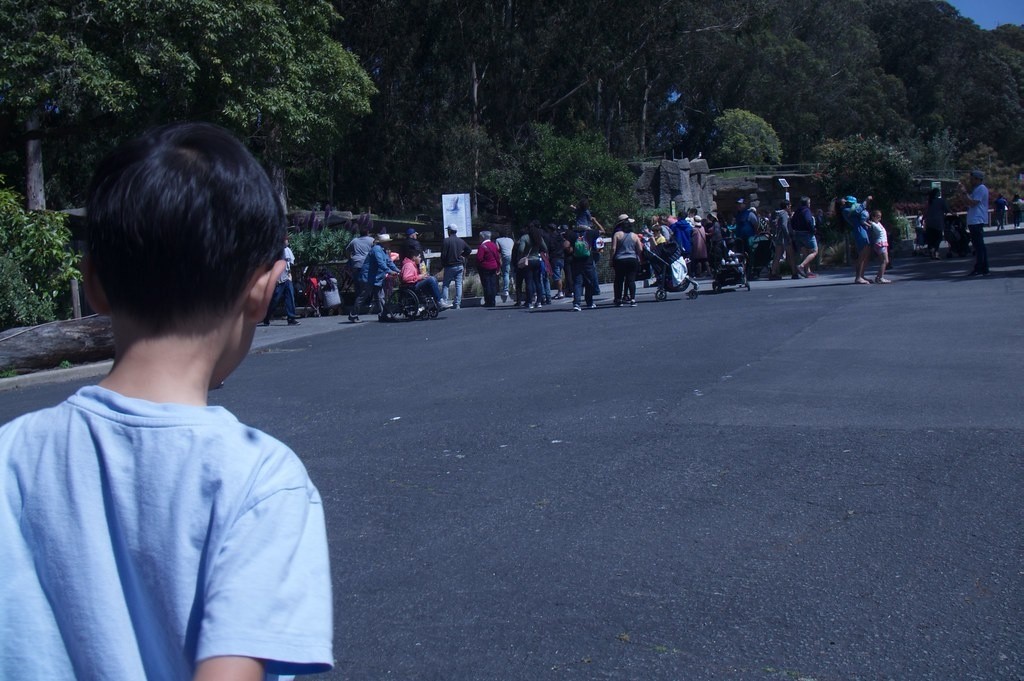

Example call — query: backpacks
[573,231,590,257]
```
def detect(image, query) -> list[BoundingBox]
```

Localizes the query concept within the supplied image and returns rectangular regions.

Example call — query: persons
[912,170,991,278]
[641,208,712,287]
[0,119,335,681]
[993,193,1009,231]
[702,206,772,281]
[401,227,426,268]
[576,198,593,231]
[609,214,643,307]
[263,239,302,326]
[1010,193,1024,228]
[400,246,453,308]
[495,227,515,296]
[441,223,472,309]
[558,216,605,311]
[512,220,564,309]
[816,195,892,284]
[476,231,501,307]
[344,226,400,322]
[769,196,818,278]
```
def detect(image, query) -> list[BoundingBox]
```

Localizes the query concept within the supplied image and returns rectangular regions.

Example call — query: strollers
[748,231,775,280]
[712,237,751,294]
[643,241,699,303]
[944,210,976,258]
[299,263,328,318]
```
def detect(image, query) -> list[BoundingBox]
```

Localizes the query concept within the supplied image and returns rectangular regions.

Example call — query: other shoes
[501,291,509,296]
[437,299,453,309]
[480,303,495,307]
[287,318,301,326]
[348,312,362,323]
[378,316,391,322]
[264,320,270,326]
[516,251,991,310]
[454,305,460,309]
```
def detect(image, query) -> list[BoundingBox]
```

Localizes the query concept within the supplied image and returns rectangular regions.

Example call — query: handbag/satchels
[563,239,573,254]
[518,257,528,268]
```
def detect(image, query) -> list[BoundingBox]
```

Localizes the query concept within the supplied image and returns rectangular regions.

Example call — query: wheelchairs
[387,273,441,322]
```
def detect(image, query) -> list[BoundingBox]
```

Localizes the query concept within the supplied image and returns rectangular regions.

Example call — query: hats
[845,196,857,204]
[545,224,556,230]
[748,207,756,211]
[559,225,568,231]
[374,233,393,243]
[615,214,635,226]
[445,224,458,231]
[690,215,702,226]
[406,229,416,235]
